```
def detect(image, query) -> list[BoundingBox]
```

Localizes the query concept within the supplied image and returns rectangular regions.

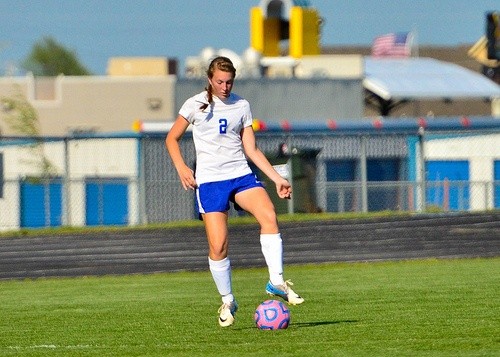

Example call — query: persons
[165,56,305,328]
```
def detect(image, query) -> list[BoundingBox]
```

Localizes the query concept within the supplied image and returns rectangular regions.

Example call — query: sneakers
[266,279,305,306]
[218,298,238,327]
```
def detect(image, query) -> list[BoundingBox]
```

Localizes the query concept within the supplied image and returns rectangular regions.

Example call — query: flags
[371,30,413,57]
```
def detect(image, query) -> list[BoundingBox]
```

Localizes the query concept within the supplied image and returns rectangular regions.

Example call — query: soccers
[255,300,289,330]
